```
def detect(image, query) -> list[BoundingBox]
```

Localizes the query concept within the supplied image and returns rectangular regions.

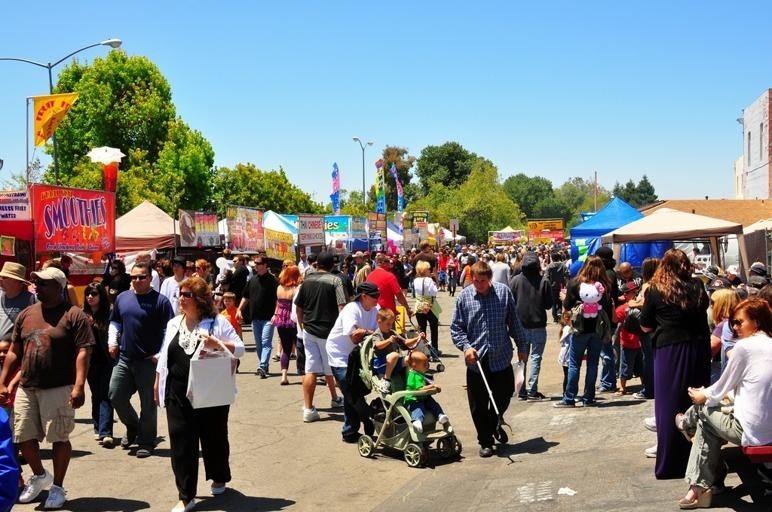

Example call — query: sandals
[678,483,713,509]
[280,379,290,385]
[675,413,693,441]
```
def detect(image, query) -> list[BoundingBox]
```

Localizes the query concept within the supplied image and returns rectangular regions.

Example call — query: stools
[709,444,771,511]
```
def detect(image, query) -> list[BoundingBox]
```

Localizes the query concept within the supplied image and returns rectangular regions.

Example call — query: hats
[30,266,67,289]
[350,281,379,301]
[0,261,31,287]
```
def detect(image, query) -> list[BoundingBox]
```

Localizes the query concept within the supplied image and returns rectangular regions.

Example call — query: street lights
[352,136,374,206]
[1,38,122,186]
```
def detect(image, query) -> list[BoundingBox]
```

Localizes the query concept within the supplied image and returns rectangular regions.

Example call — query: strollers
[356,328,463,468]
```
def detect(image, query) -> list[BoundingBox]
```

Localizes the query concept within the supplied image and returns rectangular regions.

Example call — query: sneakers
[94,433,114,448]
[210,481,226,494]
[18,468,54,503]
[330,395,345,408]
[171,498,196,512]
[44,483,66,509]
[302,405,321,422]
[643,416,657,432]
[480,424,508,458]
[342,431,363,442]
[137,438,157,458]
[120,418,141,447]
[364,420,375,436]
[515,382,655,409]
[644,444,658,458]
[254,366,269,378]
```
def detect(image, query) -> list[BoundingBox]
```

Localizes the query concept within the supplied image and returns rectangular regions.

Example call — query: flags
[32,91,81,149]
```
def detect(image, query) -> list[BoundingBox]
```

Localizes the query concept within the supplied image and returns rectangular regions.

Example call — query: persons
[0,408,23,512]
[325,282,382,443]
[675,296,771,509]
[448,261,529,458]
[151,276,246,512]
[638,246,711,482]
[402,350,448,434]
[0,266,95,509]
[0,235,772,409]
[292,251,350,424]
[81,280,118,446]
[106,262,171,457]
[641,415,660,460]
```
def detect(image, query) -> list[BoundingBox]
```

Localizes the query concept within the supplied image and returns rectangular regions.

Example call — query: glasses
[130,274,149,280]
[179,292,198,298]
[733,317,752,325]
[85,290,101,297]
